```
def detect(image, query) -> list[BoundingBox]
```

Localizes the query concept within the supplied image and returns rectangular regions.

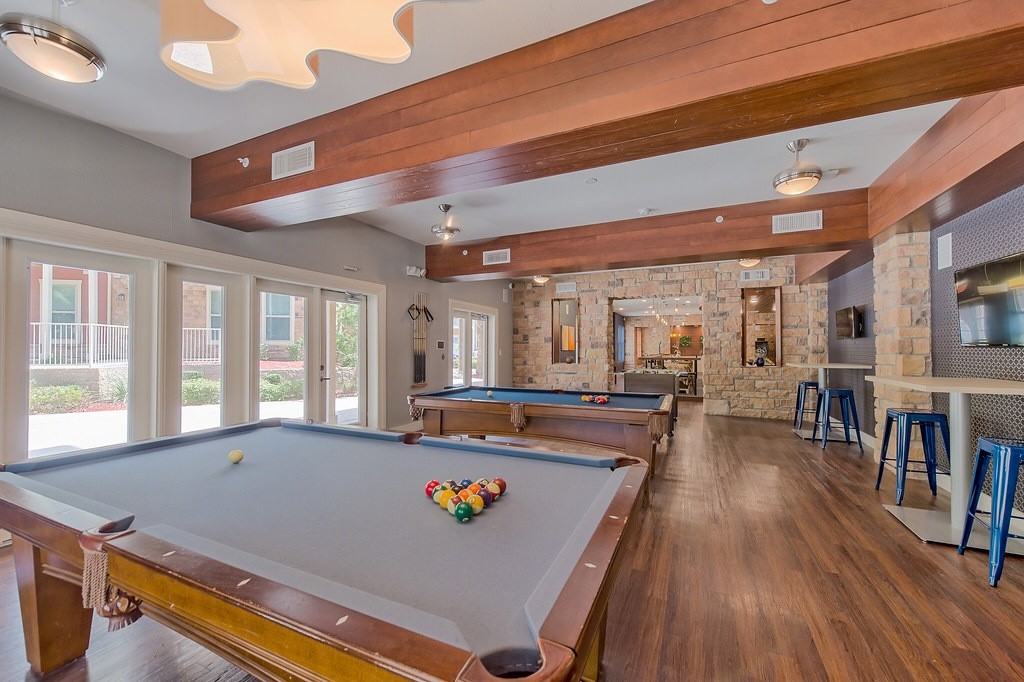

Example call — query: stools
[812,388,863,450]
[875,407,953,505]
[794,381,835,433]
[955,437,1024,588]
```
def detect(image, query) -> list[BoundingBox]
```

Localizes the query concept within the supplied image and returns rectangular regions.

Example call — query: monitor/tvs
[836,306,861,340]
[954,251,1024,348]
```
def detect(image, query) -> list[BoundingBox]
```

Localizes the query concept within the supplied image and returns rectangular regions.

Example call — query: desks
[639,355,701,395]
[785,361,873,444]
[0,415,650,682]
[864,375,1024,555]
[407,384,676,480]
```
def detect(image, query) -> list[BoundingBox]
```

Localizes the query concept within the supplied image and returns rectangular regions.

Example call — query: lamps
[533,275,551,283]
[738,257,761,268]
[772,138,822,195]
[431,203,461,240]
[0,0,108,83]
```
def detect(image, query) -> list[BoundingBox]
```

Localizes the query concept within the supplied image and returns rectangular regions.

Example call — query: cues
[412,291,429,386]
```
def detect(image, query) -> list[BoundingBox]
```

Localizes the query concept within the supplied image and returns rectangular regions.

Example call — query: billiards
[581,395,612,405]
[425,476,507,522]
[228,448,244,464]
[486,389,493,397]
